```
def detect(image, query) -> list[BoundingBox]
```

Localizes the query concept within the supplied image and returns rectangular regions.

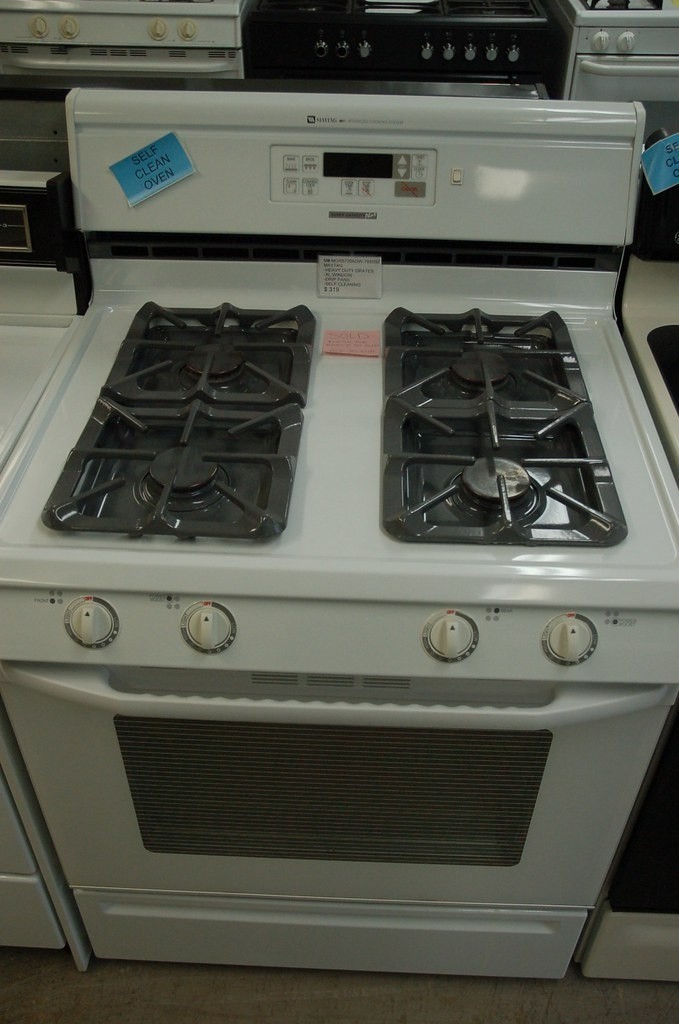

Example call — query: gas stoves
[0,93,679,658]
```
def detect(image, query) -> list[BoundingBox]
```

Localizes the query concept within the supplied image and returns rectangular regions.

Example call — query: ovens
[0,667,675,980]
